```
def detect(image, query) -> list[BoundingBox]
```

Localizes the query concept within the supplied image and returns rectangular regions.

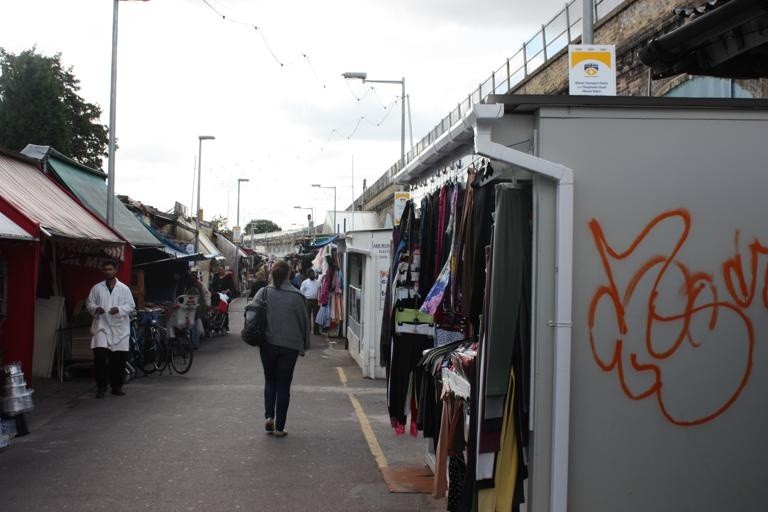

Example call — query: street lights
[106,0,152,228]
[234,178,250,289]
[312,184,336,234]
[293,206,313,228]
[193,135,216,273]
[341,72,405,162]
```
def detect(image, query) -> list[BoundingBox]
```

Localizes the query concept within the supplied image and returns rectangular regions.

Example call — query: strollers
[205,293,231,336]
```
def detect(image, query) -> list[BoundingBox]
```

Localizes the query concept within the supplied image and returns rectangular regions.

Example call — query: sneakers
[95,390,126,398]
[265,417,288,437]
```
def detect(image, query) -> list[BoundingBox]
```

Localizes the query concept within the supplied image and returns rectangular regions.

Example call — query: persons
[85,260,136,398]
[210,265,235,336]
[244,257,323,437]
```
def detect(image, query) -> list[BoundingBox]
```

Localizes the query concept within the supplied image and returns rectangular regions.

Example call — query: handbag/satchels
[240,286,268,347]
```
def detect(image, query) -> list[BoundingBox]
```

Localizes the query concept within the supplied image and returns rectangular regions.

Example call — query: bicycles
[123,305,193,384]
